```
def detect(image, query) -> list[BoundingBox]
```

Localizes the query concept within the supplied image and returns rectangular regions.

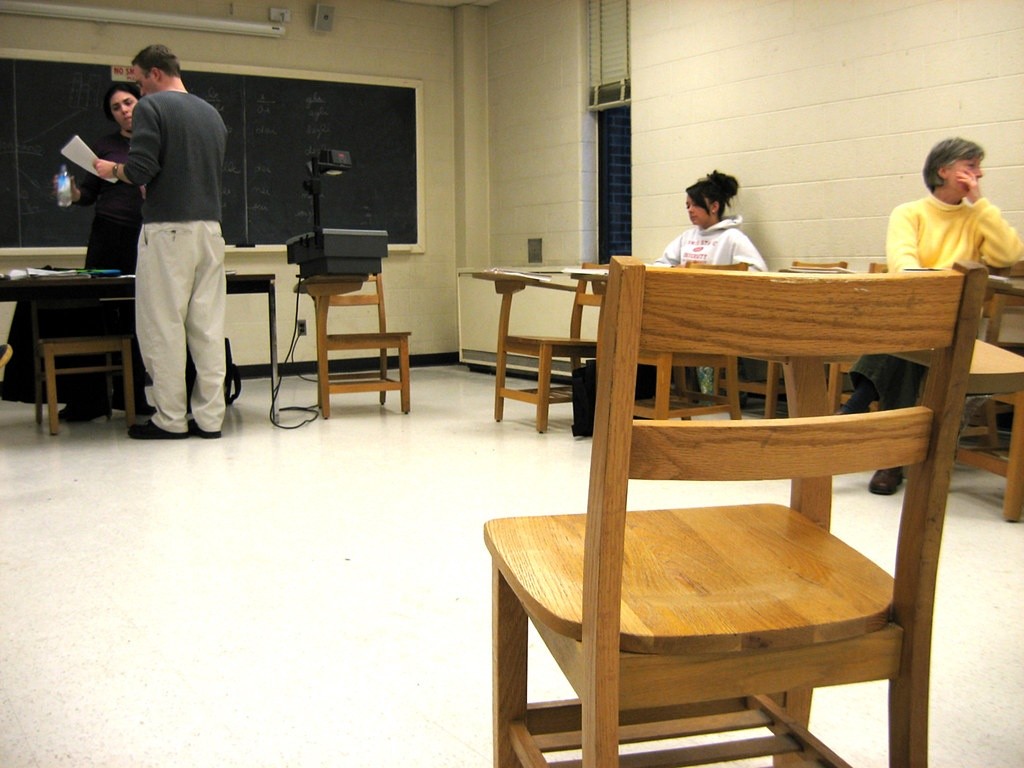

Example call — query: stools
[33,336,136,435]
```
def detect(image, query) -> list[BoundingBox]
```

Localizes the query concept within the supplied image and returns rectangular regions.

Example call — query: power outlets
[270,8,290,21]
[297,320,307,336]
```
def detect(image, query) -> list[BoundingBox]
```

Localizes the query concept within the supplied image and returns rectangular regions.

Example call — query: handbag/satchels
[185,336,241,413]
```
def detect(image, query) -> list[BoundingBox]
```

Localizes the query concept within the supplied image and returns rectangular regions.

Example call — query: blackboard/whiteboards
[0,50,429,257]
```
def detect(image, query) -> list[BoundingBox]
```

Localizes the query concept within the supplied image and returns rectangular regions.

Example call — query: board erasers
[236,240,256,248]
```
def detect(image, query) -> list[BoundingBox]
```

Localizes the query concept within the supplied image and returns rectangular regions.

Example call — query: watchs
[112,163,119,179]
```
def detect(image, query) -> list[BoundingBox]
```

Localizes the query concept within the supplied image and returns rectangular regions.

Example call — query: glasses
[135,70,150,89]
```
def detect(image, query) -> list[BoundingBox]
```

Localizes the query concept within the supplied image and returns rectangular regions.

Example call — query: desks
[571,272,608,370]
[0,274,281,424]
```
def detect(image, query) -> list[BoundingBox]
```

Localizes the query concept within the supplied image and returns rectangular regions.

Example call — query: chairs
[713,261,847,418]
[633,261,748,420]
[294,273,412,419]
[483,255,988,768]
[582,263,609,295]
[471,272,597,430]
[829,261,1024,521]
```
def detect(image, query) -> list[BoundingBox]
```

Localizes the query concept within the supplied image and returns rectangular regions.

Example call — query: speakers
[313,4,335,32]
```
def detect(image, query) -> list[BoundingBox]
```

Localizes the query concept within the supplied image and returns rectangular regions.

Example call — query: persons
[92,45,229,439]
[830,137,1024,496]
[571,170,768,440]
[52,82,143,424]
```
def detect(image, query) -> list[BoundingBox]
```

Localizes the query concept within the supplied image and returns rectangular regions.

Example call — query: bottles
[57,164,72,206]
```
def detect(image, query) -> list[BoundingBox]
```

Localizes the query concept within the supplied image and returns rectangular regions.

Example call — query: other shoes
[835,404,870,415]
[188,419,221,438]
[870,466,902,494]
[128,419,189,439]
[112,386,157,415]
